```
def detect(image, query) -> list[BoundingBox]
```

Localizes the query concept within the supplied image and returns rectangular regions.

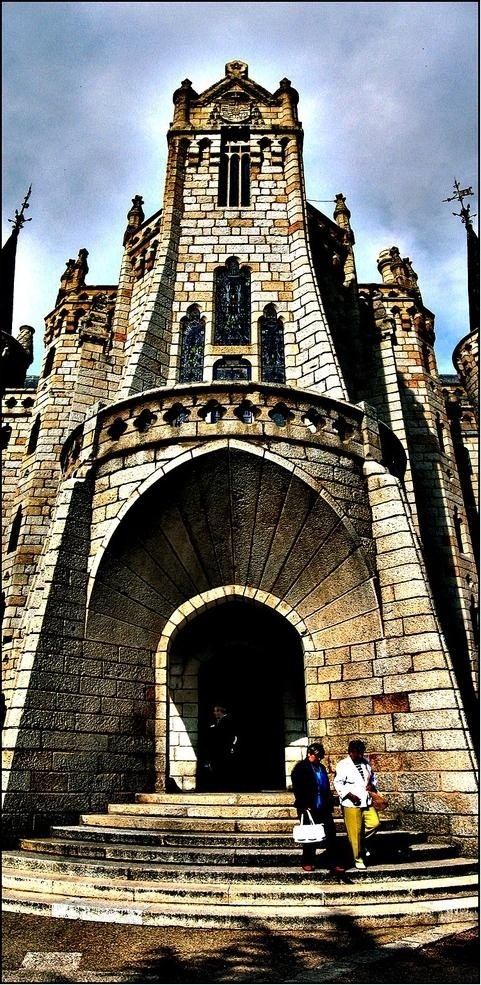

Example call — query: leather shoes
[303,865,315,871]
[332,864,346,874]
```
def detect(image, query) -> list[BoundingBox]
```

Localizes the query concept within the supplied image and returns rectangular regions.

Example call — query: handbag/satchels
[293,809,327,844]
[365,763,389,811]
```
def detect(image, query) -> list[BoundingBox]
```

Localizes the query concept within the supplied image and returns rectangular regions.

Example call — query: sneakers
[354,861,368,870]
[363,847,372,858]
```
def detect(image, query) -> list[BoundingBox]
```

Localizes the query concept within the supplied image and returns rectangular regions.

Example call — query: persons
[209,704,239,791]
[292,744,345,874]
[333,740,390,871]
